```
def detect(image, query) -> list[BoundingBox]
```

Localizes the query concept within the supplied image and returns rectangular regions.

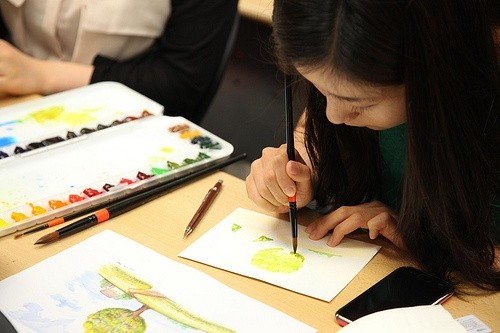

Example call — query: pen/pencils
[183,180,225,240]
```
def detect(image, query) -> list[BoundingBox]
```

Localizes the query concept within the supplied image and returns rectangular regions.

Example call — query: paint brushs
[283,65,300,255]
[14,152,246,245]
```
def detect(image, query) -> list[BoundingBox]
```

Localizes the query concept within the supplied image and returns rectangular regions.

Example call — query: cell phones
[335,266,456,327]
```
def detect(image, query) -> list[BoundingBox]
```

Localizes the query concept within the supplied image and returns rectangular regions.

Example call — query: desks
[0,171,500,333]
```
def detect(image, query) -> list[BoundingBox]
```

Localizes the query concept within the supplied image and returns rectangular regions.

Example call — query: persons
[0,1,238,126]
[241,0,499,300]
